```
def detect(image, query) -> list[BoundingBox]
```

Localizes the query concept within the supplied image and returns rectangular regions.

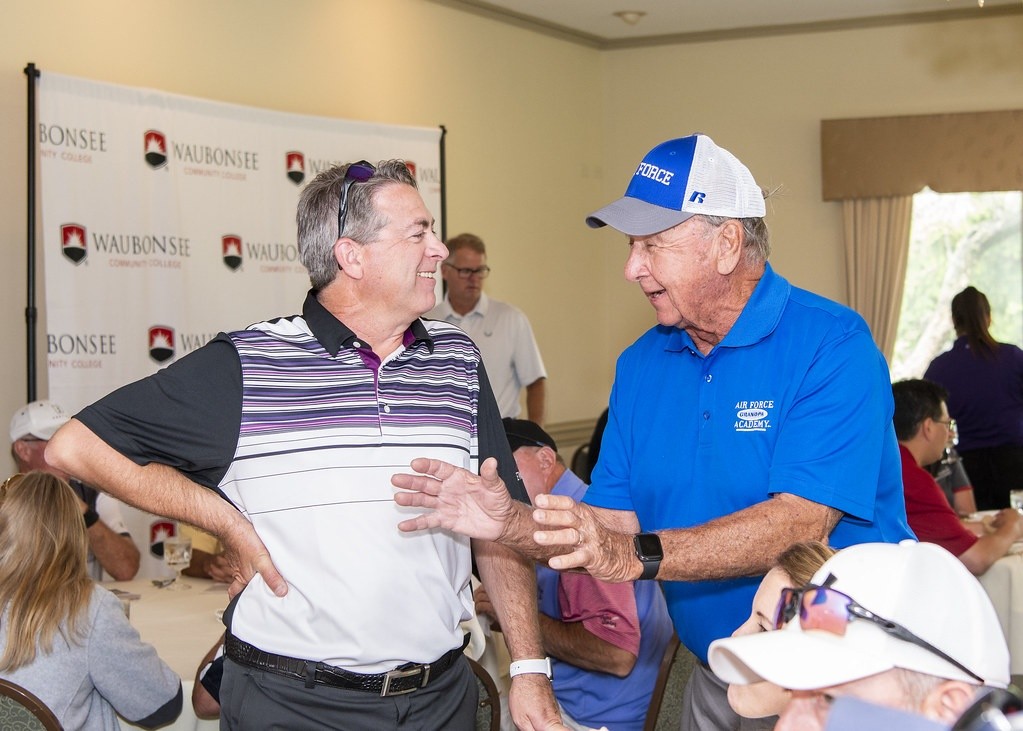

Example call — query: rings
[574,529,583,547]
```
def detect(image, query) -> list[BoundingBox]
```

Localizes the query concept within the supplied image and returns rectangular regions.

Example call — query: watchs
[633,533,663,580]
[510,656,554,681]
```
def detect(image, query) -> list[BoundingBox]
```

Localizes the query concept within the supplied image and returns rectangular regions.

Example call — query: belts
[226,631,472,697]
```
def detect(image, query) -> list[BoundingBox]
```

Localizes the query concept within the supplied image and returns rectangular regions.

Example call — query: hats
[9,401,71,441]
[585,133,767,237]
[707,539,1010,691]
[502,417,558,453]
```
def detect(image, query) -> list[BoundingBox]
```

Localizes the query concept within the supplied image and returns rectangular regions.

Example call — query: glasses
[446,263,490,279]
[337,160,377,271]
[915,418,955,431]
[774,586,983,682]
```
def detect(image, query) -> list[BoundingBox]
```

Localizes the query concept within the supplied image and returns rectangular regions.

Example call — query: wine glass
[938,417,963,465]
[163,536,192,592]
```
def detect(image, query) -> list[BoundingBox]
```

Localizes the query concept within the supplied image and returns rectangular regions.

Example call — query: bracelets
[83,505,99,529]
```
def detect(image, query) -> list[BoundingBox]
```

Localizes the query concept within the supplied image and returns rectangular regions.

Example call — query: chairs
[639,633,698,731]
[0,680,64,731]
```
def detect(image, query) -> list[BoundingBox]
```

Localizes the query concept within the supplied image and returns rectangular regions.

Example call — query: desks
[99,578,522,730]
[945,509,1023,679]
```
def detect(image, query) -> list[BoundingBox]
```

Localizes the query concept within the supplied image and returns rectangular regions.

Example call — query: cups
[1011,489,1023,514]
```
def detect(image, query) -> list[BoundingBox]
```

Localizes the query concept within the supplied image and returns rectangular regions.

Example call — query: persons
[42,158,565,731]
[0,400,232,731]
[708,539,1023,731]
[922,286,1023,511]
[891,378,1023,577]
[420,233,549,428]
[391,132,920,731]
[471,417,674,731]
